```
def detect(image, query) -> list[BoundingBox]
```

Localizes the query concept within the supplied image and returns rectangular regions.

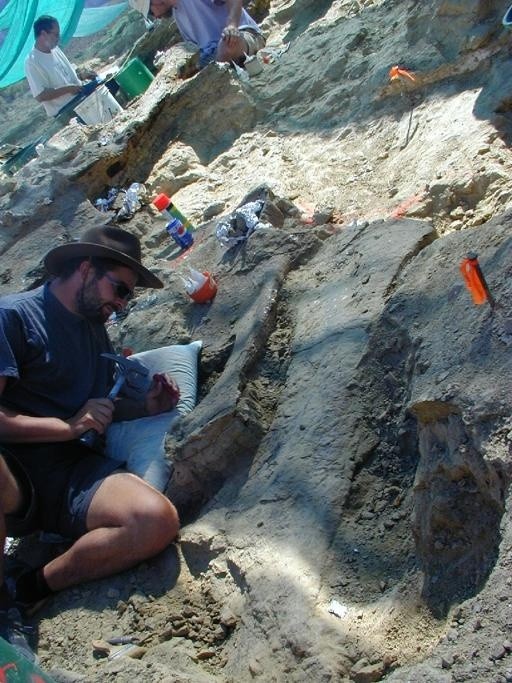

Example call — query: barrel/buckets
[72,82,124,127]
[113,58,155,99]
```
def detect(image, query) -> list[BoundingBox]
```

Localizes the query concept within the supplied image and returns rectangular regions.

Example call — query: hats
[44,224,164,289]
[127,0,151,21]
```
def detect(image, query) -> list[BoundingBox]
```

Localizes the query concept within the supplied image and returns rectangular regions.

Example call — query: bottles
[230,58,247,81]
[153,193,193,235]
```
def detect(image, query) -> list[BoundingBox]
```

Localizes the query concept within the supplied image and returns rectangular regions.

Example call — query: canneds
[165,218,194,250]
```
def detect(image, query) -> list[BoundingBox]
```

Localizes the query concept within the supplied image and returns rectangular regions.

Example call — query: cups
[243,55,264,77]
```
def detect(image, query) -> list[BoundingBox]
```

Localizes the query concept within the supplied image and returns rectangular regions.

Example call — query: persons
[131,0,268,73]
[0,225,182,607]
[23,13,99,125]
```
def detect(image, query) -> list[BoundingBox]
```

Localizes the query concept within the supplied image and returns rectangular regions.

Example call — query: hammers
[80,353,149,447]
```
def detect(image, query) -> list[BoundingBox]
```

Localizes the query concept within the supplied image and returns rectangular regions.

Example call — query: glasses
[103,273,134,302]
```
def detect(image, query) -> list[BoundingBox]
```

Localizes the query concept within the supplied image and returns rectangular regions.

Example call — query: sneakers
[1,554,42,656]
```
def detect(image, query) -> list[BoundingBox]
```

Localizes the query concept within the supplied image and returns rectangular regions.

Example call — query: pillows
[98,340,202,493]
